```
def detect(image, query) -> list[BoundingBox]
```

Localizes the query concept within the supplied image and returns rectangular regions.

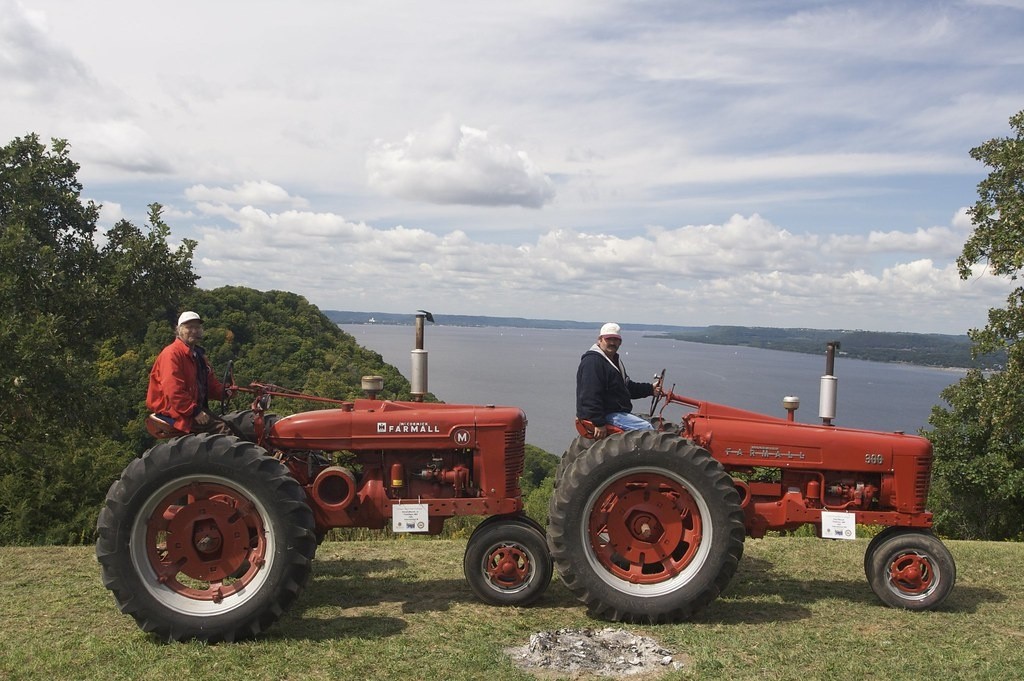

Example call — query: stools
[147,413,187,437]
[576,419,625,439]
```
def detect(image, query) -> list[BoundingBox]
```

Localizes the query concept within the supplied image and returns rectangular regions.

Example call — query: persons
[145,311,234,436]
[575,322,661,439]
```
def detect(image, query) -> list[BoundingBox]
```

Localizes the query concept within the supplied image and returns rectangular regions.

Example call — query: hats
[600,323,621,342]
[178,311,204,327]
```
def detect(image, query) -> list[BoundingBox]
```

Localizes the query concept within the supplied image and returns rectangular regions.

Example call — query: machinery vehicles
[546,338,957,627]
[95,307,555,647]
